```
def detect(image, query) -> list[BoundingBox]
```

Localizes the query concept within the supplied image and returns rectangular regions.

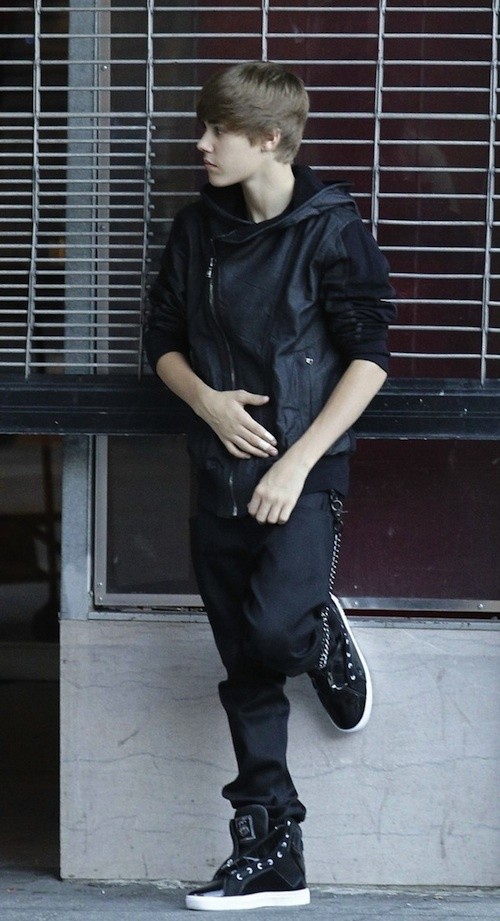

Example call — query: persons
[140,60,402,911]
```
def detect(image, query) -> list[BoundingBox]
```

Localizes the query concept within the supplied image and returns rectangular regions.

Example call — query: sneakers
[304,591,374,734]
[185,805,314,911]
